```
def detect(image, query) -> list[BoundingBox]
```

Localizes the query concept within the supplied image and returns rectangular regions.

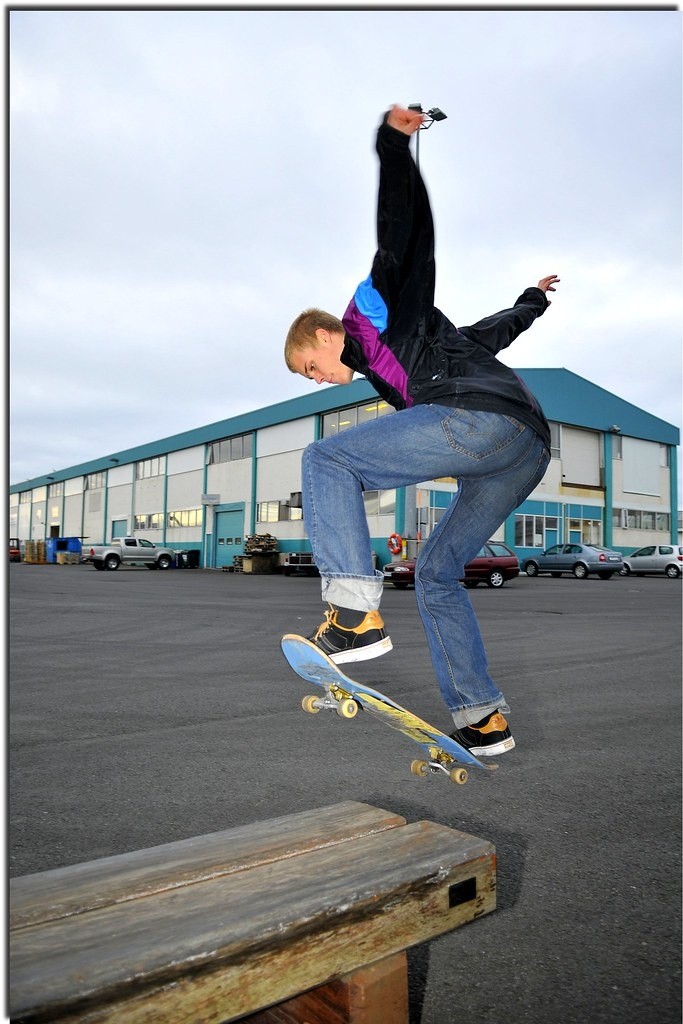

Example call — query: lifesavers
[388,533,403,555]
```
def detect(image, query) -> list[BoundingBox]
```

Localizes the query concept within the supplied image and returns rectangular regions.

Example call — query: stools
[9,799,496,1024]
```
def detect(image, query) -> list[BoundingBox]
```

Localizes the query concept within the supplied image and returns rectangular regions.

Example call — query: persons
[286,105,562,756]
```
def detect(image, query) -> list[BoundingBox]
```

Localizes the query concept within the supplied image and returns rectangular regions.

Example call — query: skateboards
[280,634,499,786]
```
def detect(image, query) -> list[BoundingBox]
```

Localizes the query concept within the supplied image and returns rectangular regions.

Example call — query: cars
[520,543,624,580]
[383,540,520,588]
[619,544,683,578]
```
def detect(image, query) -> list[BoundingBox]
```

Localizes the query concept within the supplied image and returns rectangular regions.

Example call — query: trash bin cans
[180,550,200,569]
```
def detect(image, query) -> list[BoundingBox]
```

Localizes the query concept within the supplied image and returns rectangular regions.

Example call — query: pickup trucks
[81,536,175,570]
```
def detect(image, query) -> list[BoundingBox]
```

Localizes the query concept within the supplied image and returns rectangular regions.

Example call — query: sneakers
[306,600,394,663]
[449,713,517,755]
